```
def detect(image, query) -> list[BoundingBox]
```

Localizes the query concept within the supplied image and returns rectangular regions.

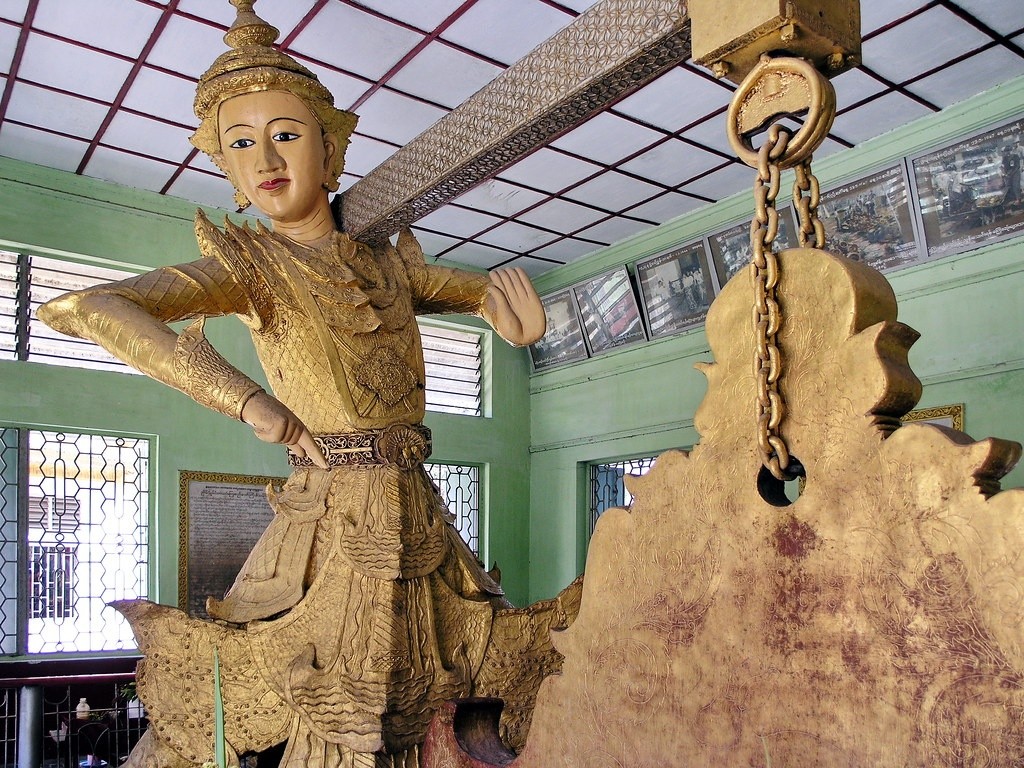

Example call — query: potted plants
[119,684,143,718]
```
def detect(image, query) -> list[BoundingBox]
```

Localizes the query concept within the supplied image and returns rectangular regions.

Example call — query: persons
[36,0,584,768]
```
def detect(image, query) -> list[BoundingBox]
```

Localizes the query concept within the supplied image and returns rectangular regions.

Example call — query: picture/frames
[571,264,648,359]
[906,110,1024,263]
[527,286,589,372]
[810,157,923,274]
[632,234,719,340]
[704,200,803,293]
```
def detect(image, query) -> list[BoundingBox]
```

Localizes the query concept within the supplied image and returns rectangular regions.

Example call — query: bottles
[75,697,91,721]
